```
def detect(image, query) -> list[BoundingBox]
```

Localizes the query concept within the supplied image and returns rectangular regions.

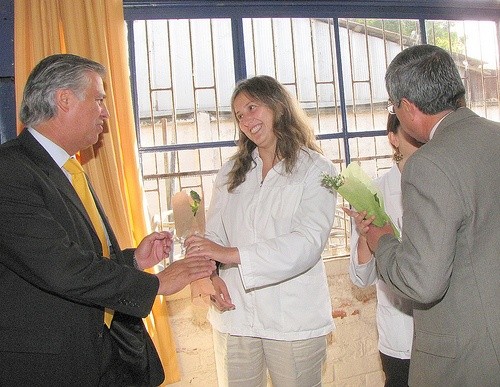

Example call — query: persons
[365,45,500,387]
[0,54,216,387]
[348,105,425,387]
[183,76,339,387]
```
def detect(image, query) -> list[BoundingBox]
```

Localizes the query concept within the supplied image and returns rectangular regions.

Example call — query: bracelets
[133,255,142,271]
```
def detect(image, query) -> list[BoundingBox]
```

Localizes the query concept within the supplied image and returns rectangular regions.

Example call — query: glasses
[385,101,402,115]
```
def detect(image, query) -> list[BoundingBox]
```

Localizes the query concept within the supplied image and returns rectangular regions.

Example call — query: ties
[63,157,116,329]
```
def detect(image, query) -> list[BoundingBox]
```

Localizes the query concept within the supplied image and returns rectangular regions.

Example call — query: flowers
[317,161,401,238]
[173,190,217,304]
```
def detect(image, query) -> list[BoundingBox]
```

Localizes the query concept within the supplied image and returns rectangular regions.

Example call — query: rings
[198,246,200,252]
